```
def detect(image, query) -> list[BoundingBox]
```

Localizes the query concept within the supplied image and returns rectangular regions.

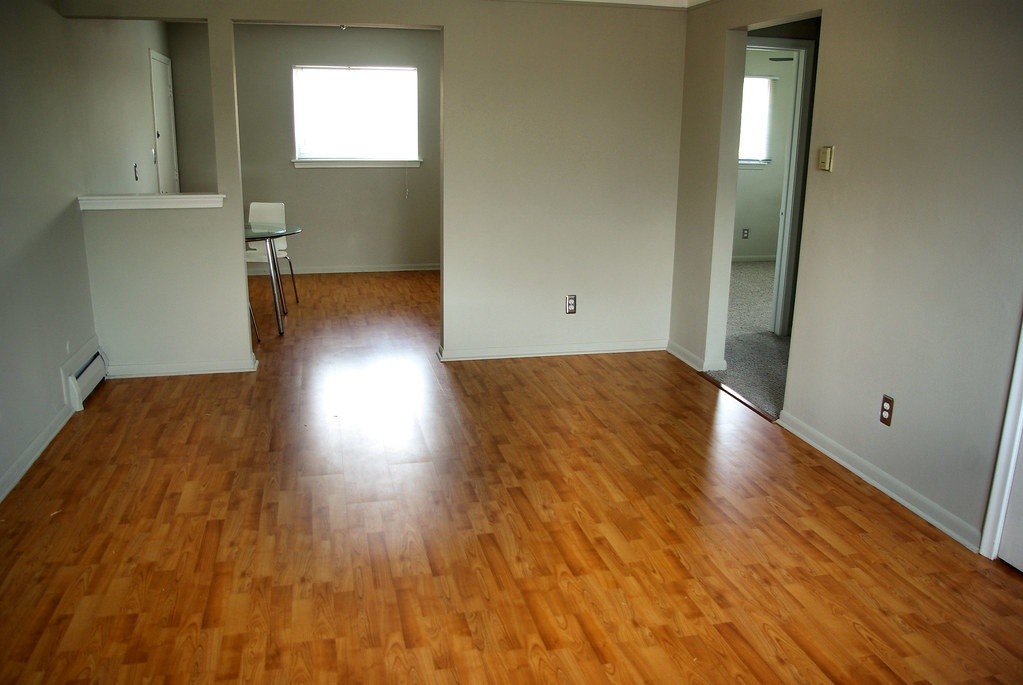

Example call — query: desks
[245,223,303,335]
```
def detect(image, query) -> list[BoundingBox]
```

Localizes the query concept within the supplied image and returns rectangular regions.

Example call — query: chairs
[246,202,299,304]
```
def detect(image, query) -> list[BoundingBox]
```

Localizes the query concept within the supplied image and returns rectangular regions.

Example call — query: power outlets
[742,229,749,239]
[880,395,894,426]
[566,295,576,314]
